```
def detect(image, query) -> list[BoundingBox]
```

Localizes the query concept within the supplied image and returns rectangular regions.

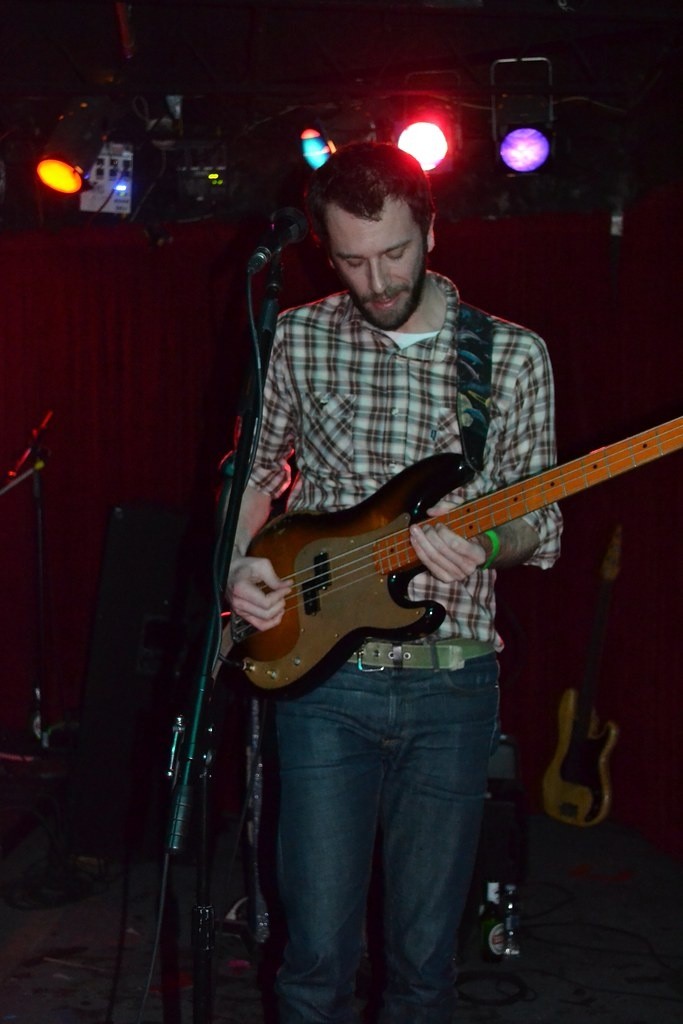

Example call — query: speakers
[64,502,235,858]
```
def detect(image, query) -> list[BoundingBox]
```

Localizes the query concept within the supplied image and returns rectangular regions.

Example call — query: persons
[216,142,565,1024]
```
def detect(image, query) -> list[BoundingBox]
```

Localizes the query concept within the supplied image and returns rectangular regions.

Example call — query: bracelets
[480,529,501,570]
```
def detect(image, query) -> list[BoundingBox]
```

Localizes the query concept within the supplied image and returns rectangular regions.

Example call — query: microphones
[247,207,309,275]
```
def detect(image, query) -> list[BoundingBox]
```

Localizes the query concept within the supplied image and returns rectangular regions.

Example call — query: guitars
[541,526,621,832]
[232,416,683,706]
